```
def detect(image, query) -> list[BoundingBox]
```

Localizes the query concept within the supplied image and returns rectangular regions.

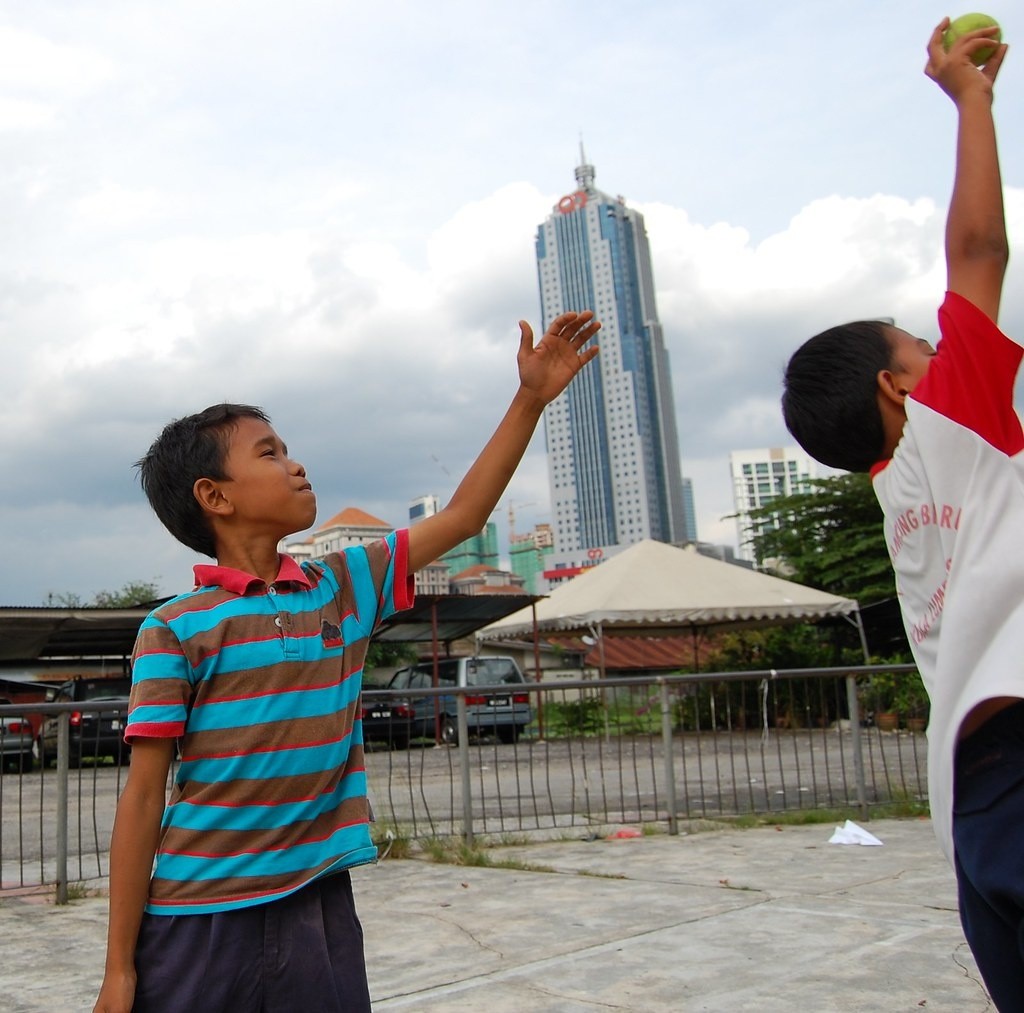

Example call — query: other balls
[942,10,1003,69]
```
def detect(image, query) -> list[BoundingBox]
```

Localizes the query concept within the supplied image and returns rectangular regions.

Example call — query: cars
[0,698,36,775]
[360,683,417,753]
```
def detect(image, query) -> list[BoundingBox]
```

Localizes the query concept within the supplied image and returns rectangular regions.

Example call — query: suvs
[35,672,132,770]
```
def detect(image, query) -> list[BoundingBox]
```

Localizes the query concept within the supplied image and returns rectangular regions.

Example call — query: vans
[386,655,534,745]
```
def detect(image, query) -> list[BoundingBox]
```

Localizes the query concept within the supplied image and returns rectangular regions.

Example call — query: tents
[472,537,875,681]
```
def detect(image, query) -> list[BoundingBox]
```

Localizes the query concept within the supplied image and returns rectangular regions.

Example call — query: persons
[781,14,1024,1013]
[90,305,602,1013]
